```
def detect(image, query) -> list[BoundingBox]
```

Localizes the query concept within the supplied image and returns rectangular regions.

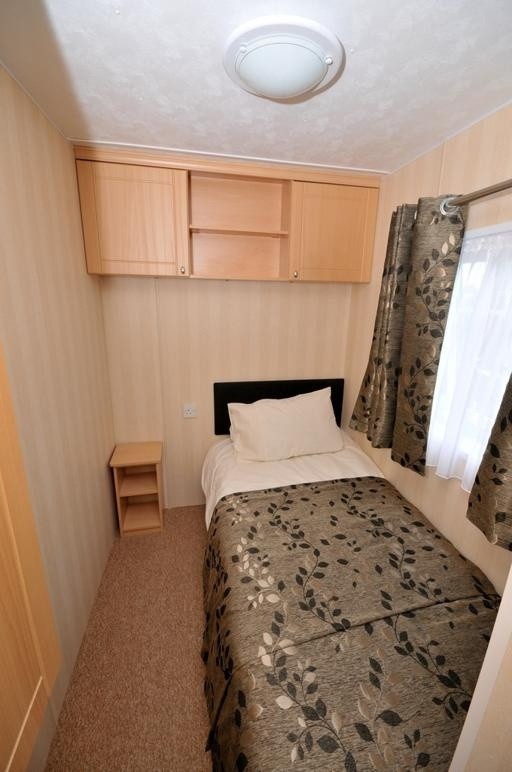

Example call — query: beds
[209,380,509,772]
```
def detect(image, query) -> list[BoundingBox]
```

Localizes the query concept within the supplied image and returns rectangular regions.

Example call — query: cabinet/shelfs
[288,180,378,282]
[75,160,192,278]
[108,442,165,541]
[190,173,287,279]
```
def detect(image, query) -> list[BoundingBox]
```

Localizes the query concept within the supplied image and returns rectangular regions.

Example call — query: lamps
[223,12,348,107]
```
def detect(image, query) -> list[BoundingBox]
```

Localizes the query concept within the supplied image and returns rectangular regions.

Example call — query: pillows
[223,385,346,464]
